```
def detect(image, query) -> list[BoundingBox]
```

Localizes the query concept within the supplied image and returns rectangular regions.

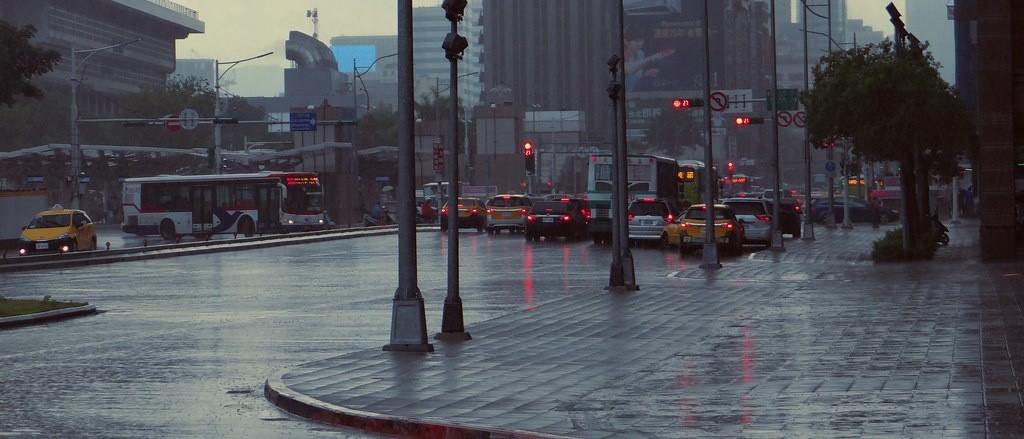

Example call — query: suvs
[722,196,778,247]
[809,193,898,225]
[628,196,674,242]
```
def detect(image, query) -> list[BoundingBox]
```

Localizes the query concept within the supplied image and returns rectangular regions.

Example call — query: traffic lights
[726,161,736,180]
[734,116,763,128]
[523,141,533,159]
[672,98,704,109]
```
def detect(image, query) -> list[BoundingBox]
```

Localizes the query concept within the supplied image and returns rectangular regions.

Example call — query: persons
[372,201,384,218]
[958,187,973,217]
[872,197,881,228]
[422,196,431,223]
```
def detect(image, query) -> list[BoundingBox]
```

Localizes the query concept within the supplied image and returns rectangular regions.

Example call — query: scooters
[922,205,951,244]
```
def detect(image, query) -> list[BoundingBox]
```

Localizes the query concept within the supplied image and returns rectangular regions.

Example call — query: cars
[19,204,98,253]
[487,194,531,235]
[667,174,823,246]
[524,201,585,243]
[442,197,486,231]
[679,202,745,252]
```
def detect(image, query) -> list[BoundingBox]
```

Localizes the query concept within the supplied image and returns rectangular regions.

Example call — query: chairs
[694,210,705,217]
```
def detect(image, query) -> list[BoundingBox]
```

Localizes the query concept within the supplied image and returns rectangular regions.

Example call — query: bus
[122,171,328,240]
[586,153,678,243]
[677,160,718,207]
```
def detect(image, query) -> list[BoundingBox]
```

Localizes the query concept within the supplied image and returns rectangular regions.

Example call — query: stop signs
[166,115,183,130]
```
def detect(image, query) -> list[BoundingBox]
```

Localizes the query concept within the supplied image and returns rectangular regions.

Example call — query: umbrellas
[870,190,887,197]
